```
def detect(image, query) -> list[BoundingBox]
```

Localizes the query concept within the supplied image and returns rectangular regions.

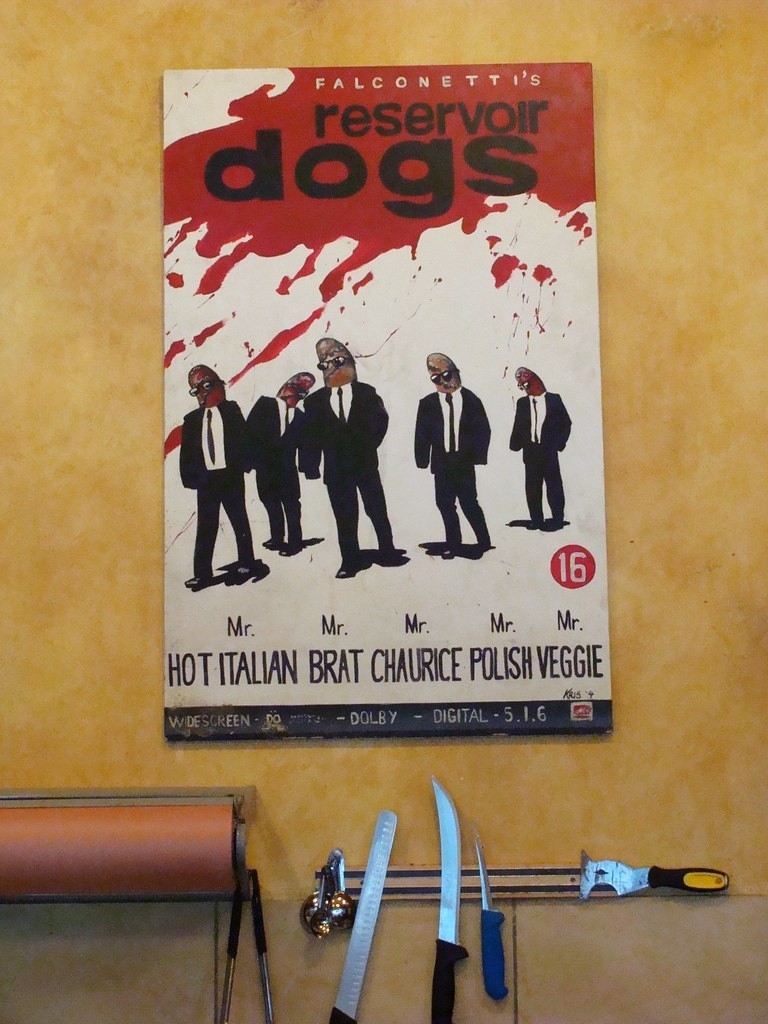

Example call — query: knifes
[424,779,471,1024]
